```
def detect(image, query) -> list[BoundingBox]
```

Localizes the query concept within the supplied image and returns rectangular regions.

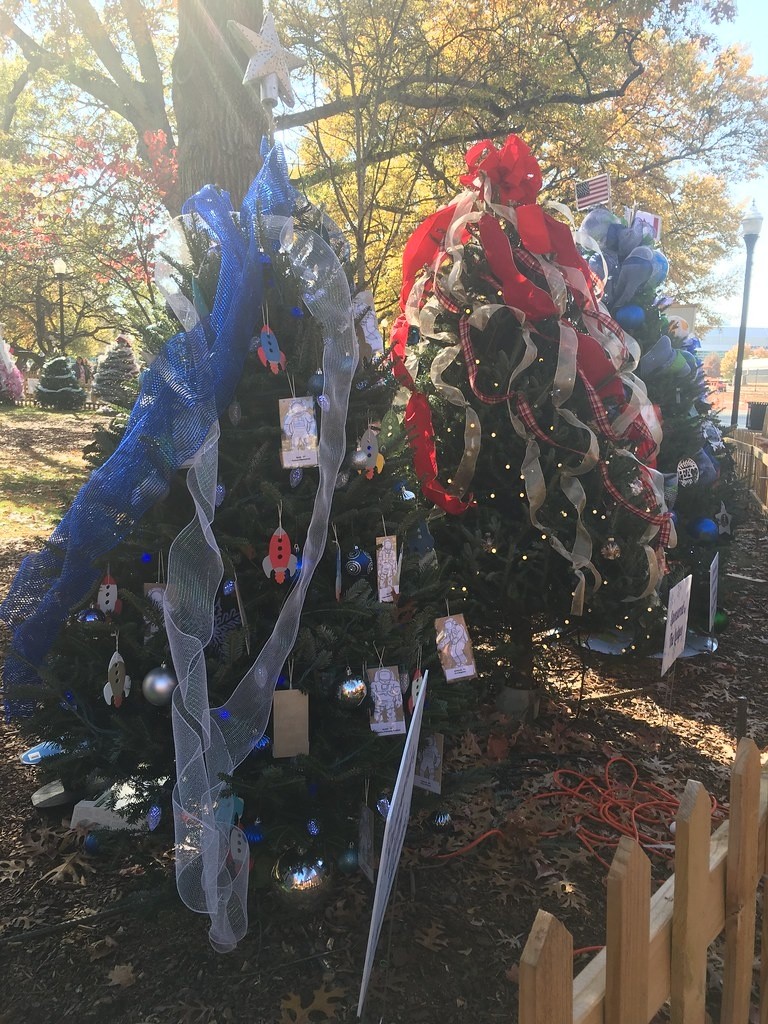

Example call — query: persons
[72,356,90,383]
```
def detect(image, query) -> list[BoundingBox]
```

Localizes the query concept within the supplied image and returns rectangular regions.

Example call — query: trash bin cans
[745,402,768,431]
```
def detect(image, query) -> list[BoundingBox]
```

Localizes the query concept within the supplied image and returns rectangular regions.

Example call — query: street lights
[53,258,68,353]
[729,199,763,428]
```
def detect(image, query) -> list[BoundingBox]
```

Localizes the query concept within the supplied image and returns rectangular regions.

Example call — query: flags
[576,175,609,209]
